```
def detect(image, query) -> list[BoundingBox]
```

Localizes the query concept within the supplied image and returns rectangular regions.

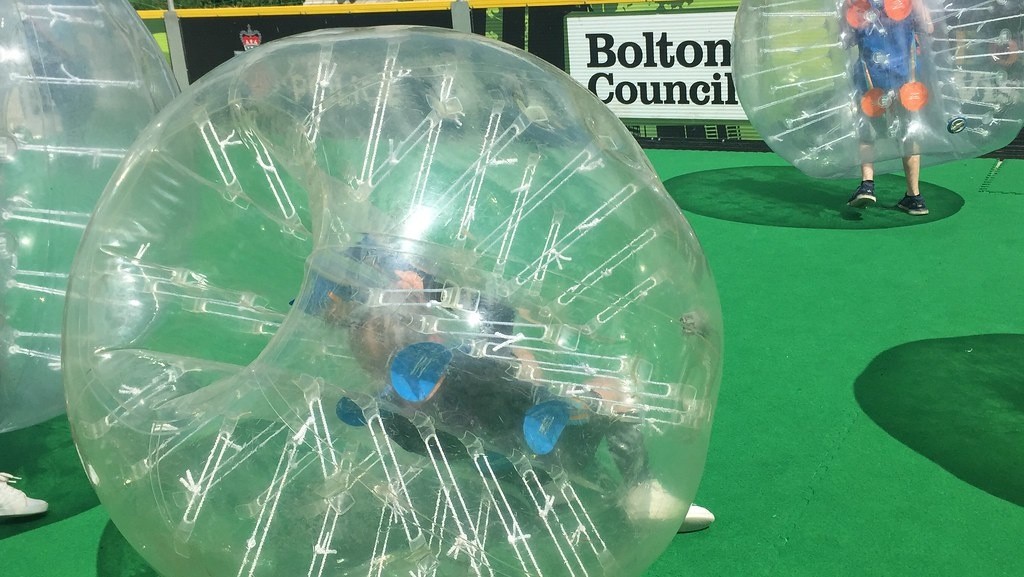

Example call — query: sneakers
[846,182,876,211]
[0,469,49,517]
[627,477,715,534]
[896,192,929,216]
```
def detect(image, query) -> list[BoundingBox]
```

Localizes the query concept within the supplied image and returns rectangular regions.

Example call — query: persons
[322,270,714,533]
[837,0,935,215]
[0,472,49,519]
